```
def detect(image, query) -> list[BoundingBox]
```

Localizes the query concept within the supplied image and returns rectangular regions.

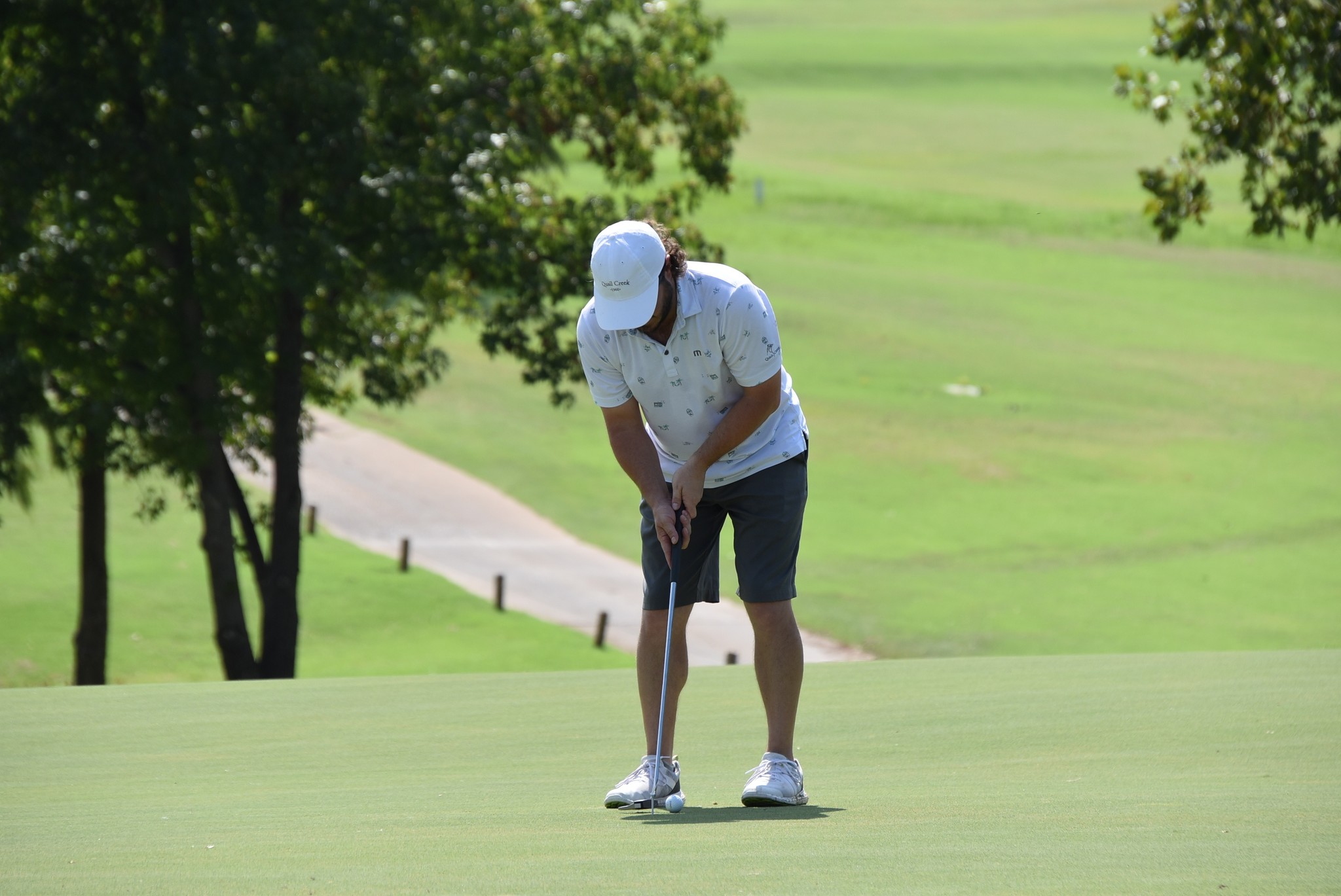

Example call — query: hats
[591,221,666,330]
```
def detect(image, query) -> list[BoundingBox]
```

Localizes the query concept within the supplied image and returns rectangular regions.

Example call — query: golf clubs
[618,501,683,811]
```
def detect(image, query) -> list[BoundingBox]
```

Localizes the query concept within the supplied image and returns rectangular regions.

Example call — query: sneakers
[741,752,809,806]
[604,755,685,809]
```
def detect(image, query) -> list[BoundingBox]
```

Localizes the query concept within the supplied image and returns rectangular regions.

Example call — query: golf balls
[665,794,683,813]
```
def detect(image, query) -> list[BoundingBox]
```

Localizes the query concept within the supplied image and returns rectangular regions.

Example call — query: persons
[575,220,811,807]
